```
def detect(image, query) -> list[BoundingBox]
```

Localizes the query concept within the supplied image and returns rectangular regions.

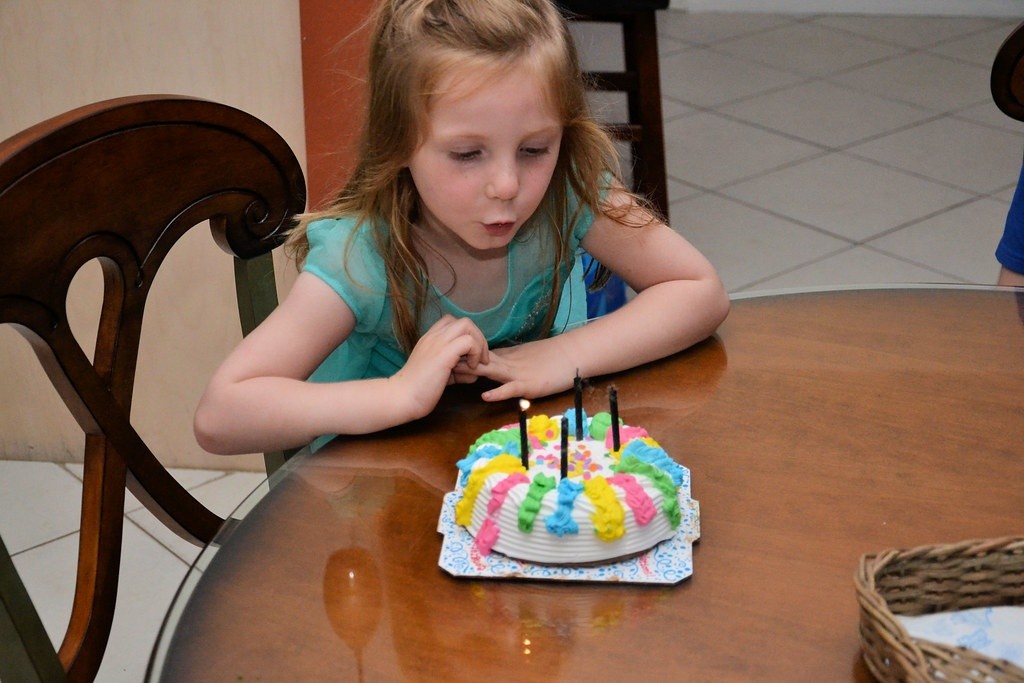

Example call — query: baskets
[855,535,1024,683]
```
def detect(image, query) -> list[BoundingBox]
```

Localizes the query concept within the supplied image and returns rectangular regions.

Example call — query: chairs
[0,94,308,683]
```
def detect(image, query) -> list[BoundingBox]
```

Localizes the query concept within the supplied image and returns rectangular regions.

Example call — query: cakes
[454,408,683,564]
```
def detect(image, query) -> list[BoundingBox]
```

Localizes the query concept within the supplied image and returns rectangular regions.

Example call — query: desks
[144,284,1024,683]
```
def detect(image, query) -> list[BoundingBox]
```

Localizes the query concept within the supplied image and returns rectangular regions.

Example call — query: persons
[280,330,729,683]
[192,0,731,456]
[995,157,1024,291]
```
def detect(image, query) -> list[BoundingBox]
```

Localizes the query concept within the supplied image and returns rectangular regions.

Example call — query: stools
[552,0,670,225]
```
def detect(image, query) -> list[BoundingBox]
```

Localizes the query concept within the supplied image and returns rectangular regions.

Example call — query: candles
[573,368,583,441]
[609,386,620,452]
[519,399,530,470]
[561,413,569,479]
